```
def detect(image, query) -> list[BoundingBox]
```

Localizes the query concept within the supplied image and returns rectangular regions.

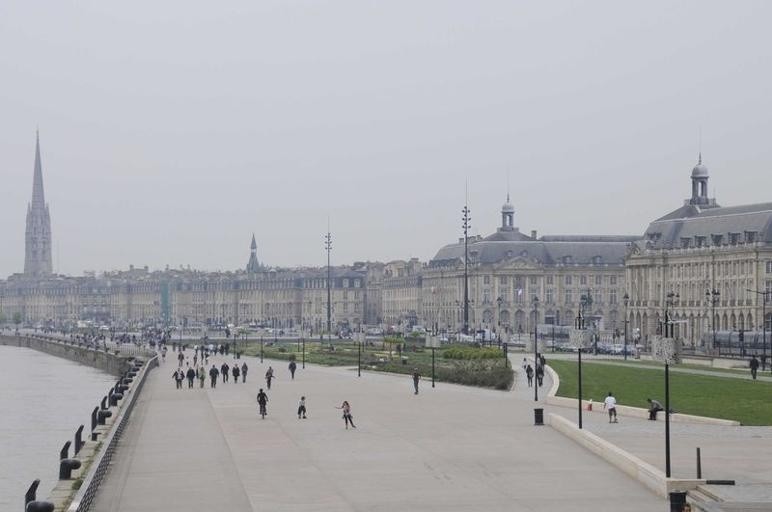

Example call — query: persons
[71,333,297,415]
[523,353,545,387]
[648,399,663,420]
[750,355,759,379]
[411,368,420,395]
[336,401,355,429]
[604,392,616,423]
[298,396,306,419]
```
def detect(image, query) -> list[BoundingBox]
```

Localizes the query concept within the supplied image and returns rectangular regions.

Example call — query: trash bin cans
[668,490,688,512]
[534,408,544,426]
[237,352,241,359]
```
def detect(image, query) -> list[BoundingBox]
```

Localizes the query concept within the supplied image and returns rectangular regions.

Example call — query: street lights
[496,296,504,350]
[532,296,541,401]
[747,289,772,354]
[622,292,630,360]
[705,287,721,350]
[461,206,470,333]
[325,234,332,334]
[667,290,680,318]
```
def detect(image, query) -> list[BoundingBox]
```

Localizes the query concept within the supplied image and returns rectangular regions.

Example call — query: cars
[549,342,635,355]
[366,324,426,337]
